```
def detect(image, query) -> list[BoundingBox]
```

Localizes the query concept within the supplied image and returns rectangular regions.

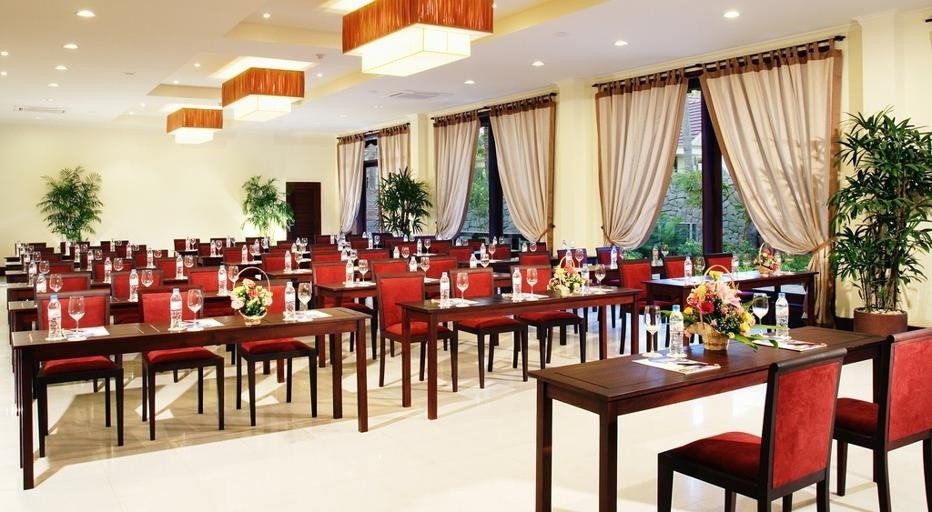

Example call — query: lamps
[222,68,304,122]
[343,0,493,77]
[167,103,223,145]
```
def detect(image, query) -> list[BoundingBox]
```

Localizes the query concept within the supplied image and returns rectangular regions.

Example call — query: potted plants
[822,103,932,336]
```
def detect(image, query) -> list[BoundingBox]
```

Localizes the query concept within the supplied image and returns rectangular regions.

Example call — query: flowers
[547,264,588,297]
[749,249,778,274]
[655,270,790,352]
[229,278,273,317]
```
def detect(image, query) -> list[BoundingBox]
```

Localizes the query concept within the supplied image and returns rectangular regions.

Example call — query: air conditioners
[646,221,661,243]
[673,222,698,243]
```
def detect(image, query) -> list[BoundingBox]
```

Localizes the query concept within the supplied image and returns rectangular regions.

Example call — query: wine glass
[695,257,705,284]
[643,306,662,358]
[421,257,430,282]
[68,296,86,338]
[19,232,627,267]
[298,284,313,321]
[742,252,752,277]
[184,256,193,276]
[359,260,368,285]
[753,293,770,335]
[187,289,203,330]
[457,273,469,308]
[784,249,794,273]
[526,268,538,301]
[594,263,605,296]
[229,266,240,293]
[661,244,669,256]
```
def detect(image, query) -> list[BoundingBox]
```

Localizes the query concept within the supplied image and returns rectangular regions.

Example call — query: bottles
[774,251,781,275]
[684,256,693,284]
[104,258,112,283]
[409,257,417,273]
[29,260,37,287]
[218,263,227,295]
[775,293,789,340]
[129,269,139,303]
[512,267,522,302]
[731,251,740,280]
[653,245,658,266]
[669,305,685,357]
[345,261,354,286]
[48,295,62,339]
[581,263,590,294]
[170,289,183,330]
[175,256,183,277]
[440,272,450,305]
[284,283,296,320]
[36,273,47,293]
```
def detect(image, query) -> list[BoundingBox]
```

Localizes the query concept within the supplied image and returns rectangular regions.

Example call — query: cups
[49,274,63,293]
[114,258,124,271]
[141,270,153,285]
[38,261,51,274]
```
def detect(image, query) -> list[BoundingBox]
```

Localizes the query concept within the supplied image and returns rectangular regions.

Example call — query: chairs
[557,247,589,334]
[188,266,238,318]
[2,234,623,297]
[445,266,529,389]
[377,272,458,393]
[370,259,410,356]
[509,264,587,370]
[662,255,703,344]
[657,347,848,510]
[421,255,459,351]
[828,325,927,509]
[34,289,125,458]
[110,269,166,323]
[310,261,377,362]
[135,287,224,441]
[229,279,322,427]
[615,257,672,354]
[595,244,623,329]
[704,252,754,302]
[518,251,553,338]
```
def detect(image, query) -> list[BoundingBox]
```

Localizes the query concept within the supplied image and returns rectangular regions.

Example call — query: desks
[640,267,820,352]
[7,286,321,415]
[529,326,897,512]
[395,285,647,421]
[10,307,373,490]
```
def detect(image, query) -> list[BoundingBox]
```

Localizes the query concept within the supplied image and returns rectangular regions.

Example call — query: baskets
[233,267,271,326]
[550,256,575,297]
[755,242,776,273]
[687,265,730,351]
